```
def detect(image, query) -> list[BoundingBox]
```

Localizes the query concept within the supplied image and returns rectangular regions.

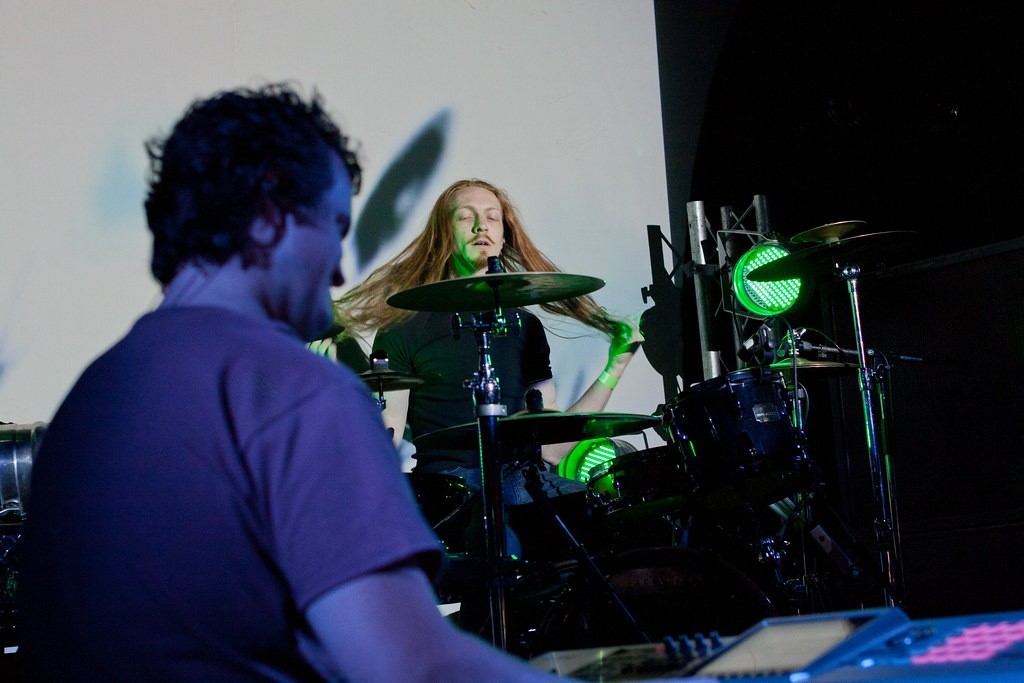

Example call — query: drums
[591,447,694,512]
[667,362,816,498]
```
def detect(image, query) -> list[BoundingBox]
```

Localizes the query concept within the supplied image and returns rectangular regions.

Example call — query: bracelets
[598,369,619,390]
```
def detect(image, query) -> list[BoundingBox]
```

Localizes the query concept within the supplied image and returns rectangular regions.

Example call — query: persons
[15,82,587,683]
[322,178,644,578]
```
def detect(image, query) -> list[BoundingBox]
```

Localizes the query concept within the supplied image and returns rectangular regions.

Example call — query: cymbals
[356,372,430,398]
[749,359,852,371]
[386,270,607,309]
[744,216,913,281]
[412,408,662,447]
